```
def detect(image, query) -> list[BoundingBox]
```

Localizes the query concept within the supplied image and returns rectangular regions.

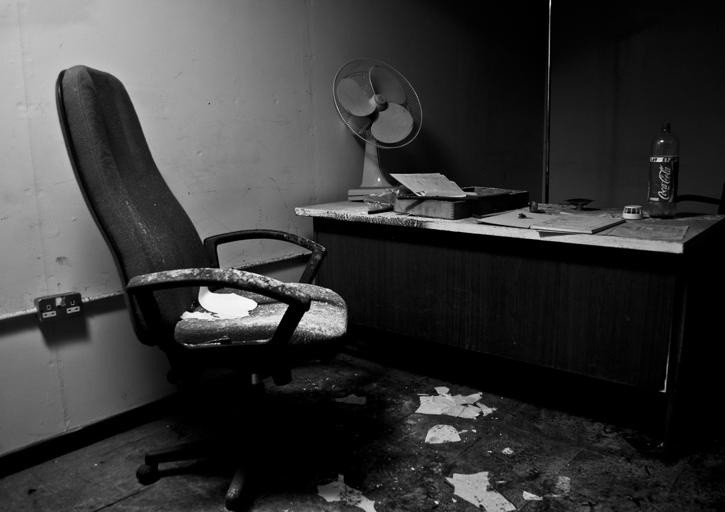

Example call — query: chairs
[58,66,348,512]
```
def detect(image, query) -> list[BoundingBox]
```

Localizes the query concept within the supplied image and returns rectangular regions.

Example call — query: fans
[333,57,423,202]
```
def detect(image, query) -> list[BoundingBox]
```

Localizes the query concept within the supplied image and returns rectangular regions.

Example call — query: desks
[294,200,724,447]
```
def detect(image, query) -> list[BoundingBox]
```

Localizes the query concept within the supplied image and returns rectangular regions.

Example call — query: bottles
[646,121,678,217]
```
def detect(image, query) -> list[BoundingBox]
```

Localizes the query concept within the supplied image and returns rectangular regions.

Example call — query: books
[388,173,477,197]
[529,213,624,233]
